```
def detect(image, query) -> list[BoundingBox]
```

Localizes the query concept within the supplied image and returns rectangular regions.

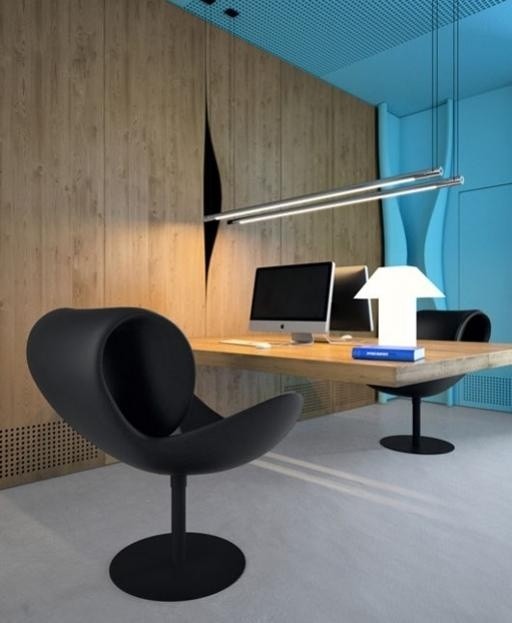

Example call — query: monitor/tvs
[248,260,336,344]
[313,265,375,343]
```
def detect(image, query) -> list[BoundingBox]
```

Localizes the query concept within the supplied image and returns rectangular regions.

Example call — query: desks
[175,331,510,417]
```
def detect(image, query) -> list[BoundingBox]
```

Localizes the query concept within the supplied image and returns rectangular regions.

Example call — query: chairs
[363,308,491,456]
[27,305,302,602]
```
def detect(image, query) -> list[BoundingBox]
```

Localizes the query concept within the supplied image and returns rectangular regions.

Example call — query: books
[350,344,425,362]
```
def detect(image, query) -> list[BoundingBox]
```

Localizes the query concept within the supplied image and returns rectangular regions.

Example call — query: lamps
[354,264,446,348]
